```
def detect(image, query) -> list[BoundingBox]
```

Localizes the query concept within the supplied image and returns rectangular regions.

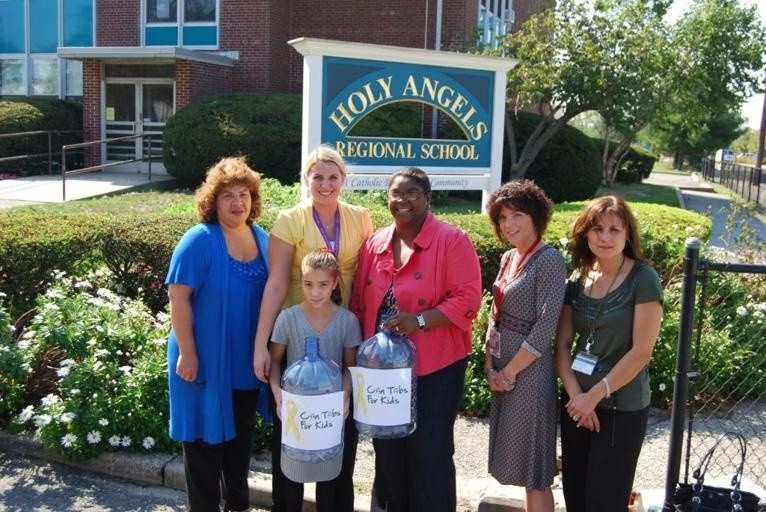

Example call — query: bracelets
[485,367,494,378]
[602,376,612,399]
[416,312,426,331]
[499,368,517,391]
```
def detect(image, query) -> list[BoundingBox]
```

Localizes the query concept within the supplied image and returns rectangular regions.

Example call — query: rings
[572,415,579,422]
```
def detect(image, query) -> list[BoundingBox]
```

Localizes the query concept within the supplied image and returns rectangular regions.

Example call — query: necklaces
[318,217,336,231]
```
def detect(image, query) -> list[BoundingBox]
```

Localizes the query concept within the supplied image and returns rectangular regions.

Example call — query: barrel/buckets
[356,313,419,441]
[280,336,345,484]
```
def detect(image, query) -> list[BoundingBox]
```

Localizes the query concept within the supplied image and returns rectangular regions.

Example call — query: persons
[554,194,667,512]
[348,167,484,511]
[164,152,274,510]
[482,179,569,512]
[267,246,364,511]
[252,144,374,511]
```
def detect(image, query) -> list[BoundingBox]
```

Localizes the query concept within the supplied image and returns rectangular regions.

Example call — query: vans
[716,149,736,165]
